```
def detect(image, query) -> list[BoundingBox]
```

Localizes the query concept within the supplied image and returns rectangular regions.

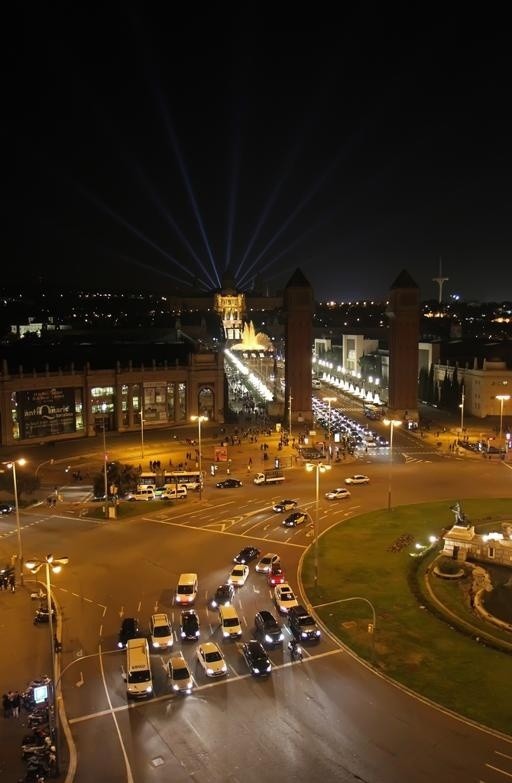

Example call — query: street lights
[496,395,511,449]
[191,416,208,472]
[318,360,384,406]
[2,458,27,584]
[305,461,331,575]
[383,419,402,508]
[26,554,68,665]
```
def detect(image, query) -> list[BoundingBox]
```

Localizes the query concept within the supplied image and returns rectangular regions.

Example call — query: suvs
[255,610,284,646]
[275,585,321,642]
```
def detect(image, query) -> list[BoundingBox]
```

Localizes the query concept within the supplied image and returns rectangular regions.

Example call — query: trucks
[254,471,285,485]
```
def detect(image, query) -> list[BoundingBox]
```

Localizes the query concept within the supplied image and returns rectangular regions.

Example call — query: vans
[149,614,173,650]
[126,487,188,502]
[116,617,154,701]
[176,573,199,606]
[218,607,242,639]
[312,379,321,390]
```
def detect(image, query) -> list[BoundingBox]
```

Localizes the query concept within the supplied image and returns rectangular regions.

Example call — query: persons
[148,458,193,472]
[231,396,275,443]
[418,420,494,461]
[0,565,17,593]
[2,690,24,719]
[73,470,83,481]
[186,426,235,476]
[248,443,269,471]
[277,427,309,450]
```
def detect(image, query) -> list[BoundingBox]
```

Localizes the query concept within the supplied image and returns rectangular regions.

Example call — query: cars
[312,395,389,448]
[179,609,200,641]
[216,478,242,488]
[242,640,272,678]
[227,565,249,587]
[345,474,369,485]
[196,642,227,677]
[234,546,259,565]
[212,583,234,609]
[282,512,307,528]
[0,503,12,515]
[273,500,297,513]
[167,657,193,695]
[324,488,350,500]
[254,552,286,587]
[403,419,418,430]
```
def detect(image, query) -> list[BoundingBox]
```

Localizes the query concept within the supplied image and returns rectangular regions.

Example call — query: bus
[136,471,204,492]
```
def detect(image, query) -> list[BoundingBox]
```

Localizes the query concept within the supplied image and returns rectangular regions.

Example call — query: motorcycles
[288,639,303,661]
[31,587,47,601]
[20,675,56,783]
[33,605,56,625]
